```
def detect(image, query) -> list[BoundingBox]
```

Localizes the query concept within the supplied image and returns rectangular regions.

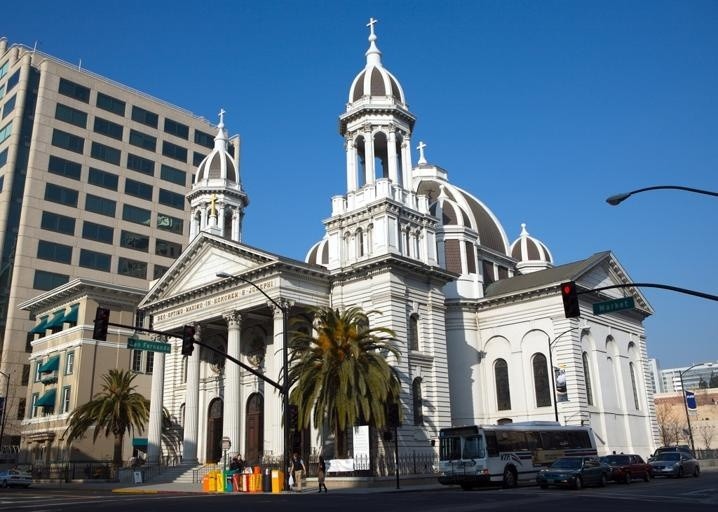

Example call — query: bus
[427,417,599,492]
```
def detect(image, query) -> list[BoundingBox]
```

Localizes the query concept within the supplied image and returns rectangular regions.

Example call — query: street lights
[603,184,718,207]
[215,269,290,493]
[545,326,592,426]
[677,360,713,464]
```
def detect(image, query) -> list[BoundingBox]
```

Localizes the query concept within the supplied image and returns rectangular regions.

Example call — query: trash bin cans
[134,471,143,484]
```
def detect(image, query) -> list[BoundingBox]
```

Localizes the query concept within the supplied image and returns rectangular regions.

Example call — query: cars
[532,442,701,494]
[0,469,33,490]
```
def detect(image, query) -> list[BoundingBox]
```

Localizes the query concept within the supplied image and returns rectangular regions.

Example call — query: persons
[290,452,308,492]
[316,454,328,493]
[229,454,253,474]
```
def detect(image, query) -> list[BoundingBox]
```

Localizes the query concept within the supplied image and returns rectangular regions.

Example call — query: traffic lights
[181,324,195,357]
[92,306,109,342]
[559,280,581,319]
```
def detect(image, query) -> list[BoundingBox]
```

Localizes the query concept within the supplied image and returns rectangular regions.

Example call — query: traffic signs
[592,295,634,317]
[124,338,170,353]
